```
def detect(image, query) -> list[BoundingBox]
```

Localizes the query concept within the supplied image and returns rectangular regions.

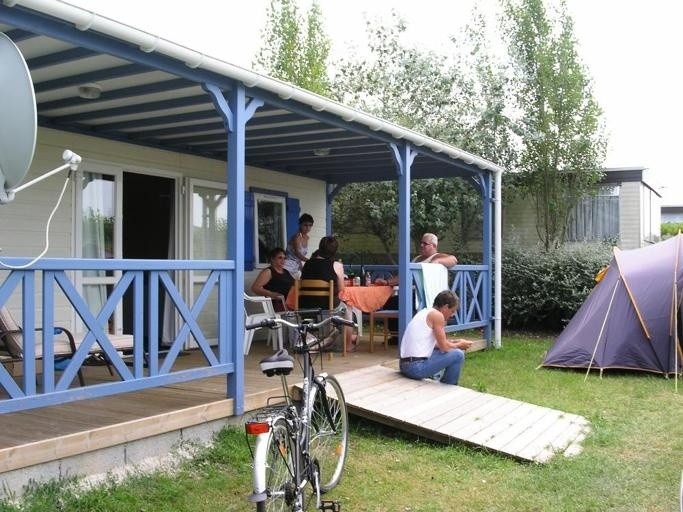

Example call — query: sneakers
[351,335,358,346]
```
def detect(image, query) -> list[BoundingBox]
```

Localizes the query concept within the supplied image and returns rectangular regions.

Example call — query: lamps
[78,85,102,100]
[313,149,329,156]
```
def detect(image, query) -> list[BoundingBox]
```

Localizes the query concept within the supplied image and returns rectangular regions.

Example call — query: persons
[374,233,458,286]
[399,290,473,385]
[285,214,314,282]
[252,247,295,313]
[298,237,360,353]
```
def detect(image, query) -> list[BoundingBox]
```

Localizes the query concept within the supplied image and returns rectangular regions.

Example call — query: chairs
[369,311,398,353]
[266,295,293,351]
[243,292,283,355]
[0,305,148,388]
[294,279,349,361]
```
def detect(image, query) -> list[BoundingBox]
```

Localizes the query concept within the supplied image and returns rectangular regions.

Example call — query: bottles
[360,263,365,286]
[365,271,372,286]
[338,258,344,264]
[347,263,355,279]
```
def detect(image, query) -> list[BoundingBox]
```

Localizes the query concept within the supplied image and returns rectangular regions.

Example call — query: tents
[536,229,683,393]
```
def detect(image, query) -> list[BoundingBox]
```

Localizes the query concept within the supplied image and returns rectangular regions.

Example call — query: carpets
[147,351,192,359]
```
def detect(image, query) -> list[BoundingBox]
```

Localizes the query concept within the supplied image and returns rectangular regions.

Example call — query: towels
[414,262,449,310]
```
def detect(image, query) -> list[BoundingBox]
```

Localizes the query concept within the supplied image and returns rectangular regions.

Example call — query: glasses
[419,242,432,246]
[302,223,313,227]
[273,256,287,260]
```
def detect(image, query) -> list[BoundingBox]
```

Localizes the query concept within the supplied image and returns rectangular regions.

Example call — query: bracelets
[386,279,388,285]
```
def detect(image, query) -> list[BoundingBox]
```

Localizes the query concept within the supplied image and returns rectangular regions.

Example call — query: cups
[353,276,361,286]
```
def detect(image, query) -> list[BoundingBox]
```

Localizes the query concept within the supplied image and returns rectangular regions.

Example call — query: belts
[399,357,427,363]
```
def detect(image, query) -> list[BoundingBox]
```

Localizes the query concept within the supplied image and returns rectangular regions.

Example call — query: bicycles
[244,315,360,512]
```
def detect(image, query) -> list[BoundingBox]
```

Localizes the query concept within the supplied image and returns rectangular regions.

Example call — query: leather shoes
[382,338,397,345]
